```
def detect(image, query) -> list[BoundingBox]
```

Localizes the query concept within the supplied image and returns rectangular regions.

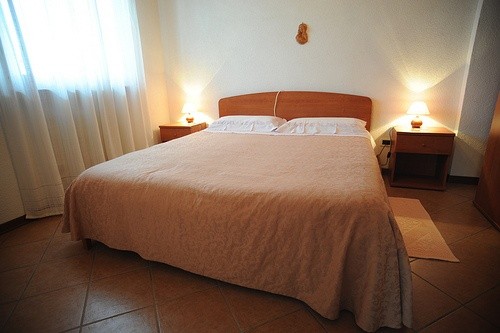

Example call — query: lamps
[182,103,196,123]
[409,101,429,127]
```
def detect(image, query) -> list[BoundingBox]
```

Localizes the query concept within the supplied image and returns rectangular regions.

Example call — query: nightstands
[389,126,456,191]
[158,119,206,143]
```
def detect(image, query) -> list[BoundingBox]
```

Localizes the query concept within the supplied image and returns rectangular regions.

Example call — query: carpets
[388,197,459,263]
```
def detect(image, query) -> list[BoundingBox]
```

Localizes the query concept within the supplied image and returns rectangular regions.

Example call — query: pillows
[202,113,288,136]
[272,117,373,141]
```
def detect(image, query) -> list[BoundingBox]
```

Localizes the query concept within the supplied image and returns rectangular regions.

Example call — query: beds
[60,90,414,331]
